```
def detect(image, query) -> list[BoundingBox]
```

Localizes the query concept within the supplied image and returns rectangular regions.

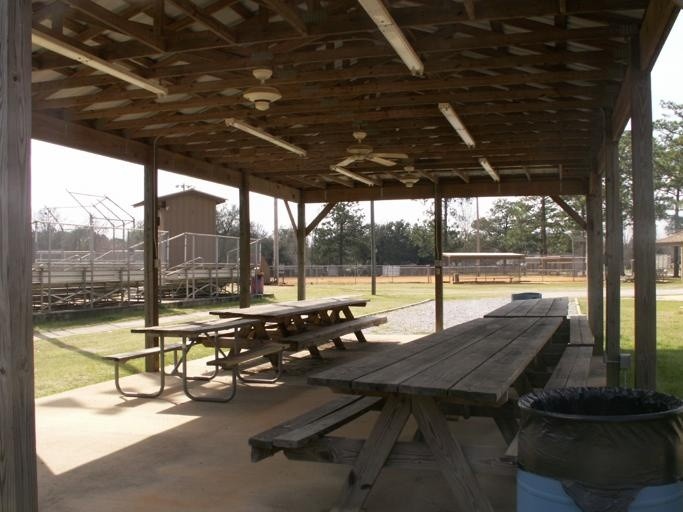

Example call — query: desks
[132,295,371,401]
[308,271,569,512]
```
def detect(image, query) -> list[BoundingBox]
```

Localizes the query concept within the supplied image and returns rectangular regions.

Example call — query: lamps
[31,28,168,97]
[357,1,476,147]
[226,117,307,157]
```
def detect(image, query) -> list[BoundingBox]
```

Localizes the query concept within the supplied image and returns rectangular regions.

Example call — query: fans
[336,132,408,168]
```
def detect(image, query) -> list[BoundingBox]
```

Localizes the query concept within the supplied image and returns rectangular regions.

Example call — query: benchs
[103,341,192,398]
[503,314,595,463]
[248,393,382,511]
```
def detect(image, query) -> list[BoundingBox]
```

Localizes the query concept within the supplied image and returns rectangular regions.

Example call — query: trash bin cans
[515,386,683,511]
[453,273,459,282]
[251,272,264,294]
